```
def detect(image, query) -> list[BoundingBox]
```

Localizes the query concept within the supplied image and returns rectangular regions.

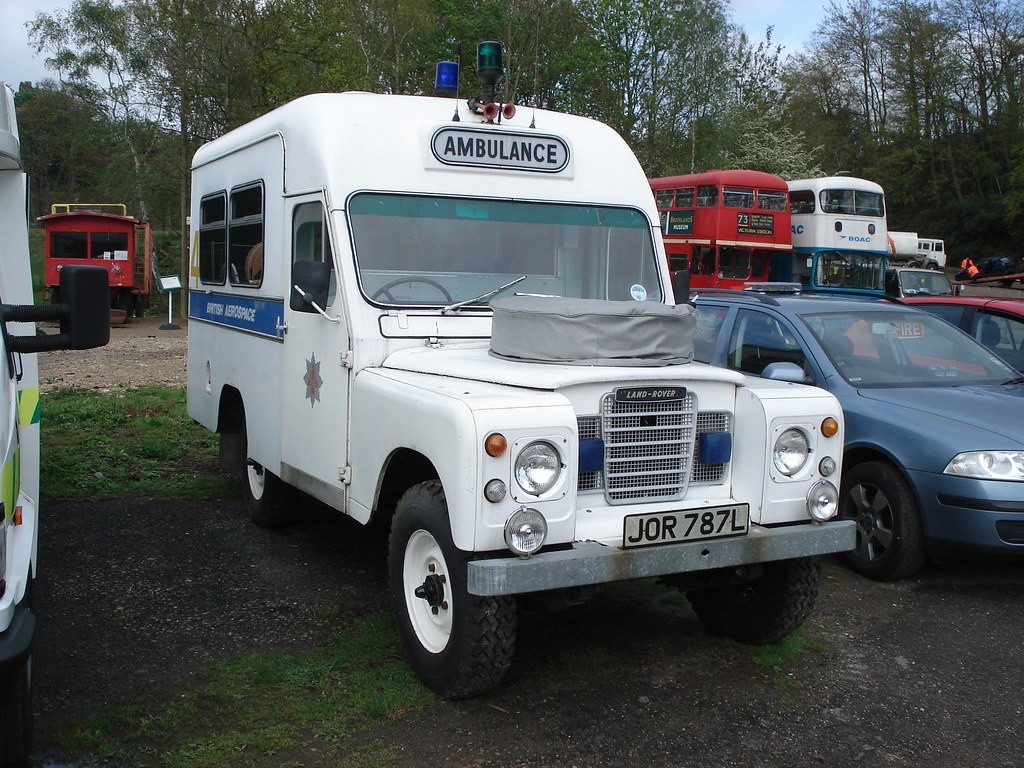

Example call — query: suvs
[688,286,1024,582]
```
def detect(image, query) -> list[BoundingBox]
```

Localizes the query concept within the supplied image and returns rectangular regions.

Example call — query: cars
[878,293,1024,376]
[887,266,961,299]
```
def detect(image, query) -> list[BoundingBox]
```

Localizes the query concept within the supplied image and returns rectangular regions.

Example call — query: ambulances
[187,89,857,704]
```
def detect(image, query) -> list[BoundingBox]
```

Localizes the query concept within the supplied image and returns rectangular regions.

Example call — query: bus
[647,169,793,294]
[785,176,889,301]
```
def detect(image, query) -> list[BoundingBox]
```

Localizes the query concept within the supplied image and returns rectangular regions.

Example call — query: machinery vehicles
[888,231,947,270]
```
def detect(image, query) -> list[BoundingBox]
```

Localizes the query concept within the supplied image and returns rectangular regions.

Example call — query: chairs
[981,320,1002,358]
[741,333,791,375]
[822,332,854,365]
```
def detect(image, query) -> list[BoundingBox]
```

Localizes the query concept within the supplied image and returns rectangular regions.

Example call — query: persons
[961,256,983,284]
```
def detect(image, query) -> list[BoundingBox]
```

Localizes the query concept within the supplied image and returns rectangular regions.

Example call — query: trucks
[0,80,112,767]
[37,203,153,324]
